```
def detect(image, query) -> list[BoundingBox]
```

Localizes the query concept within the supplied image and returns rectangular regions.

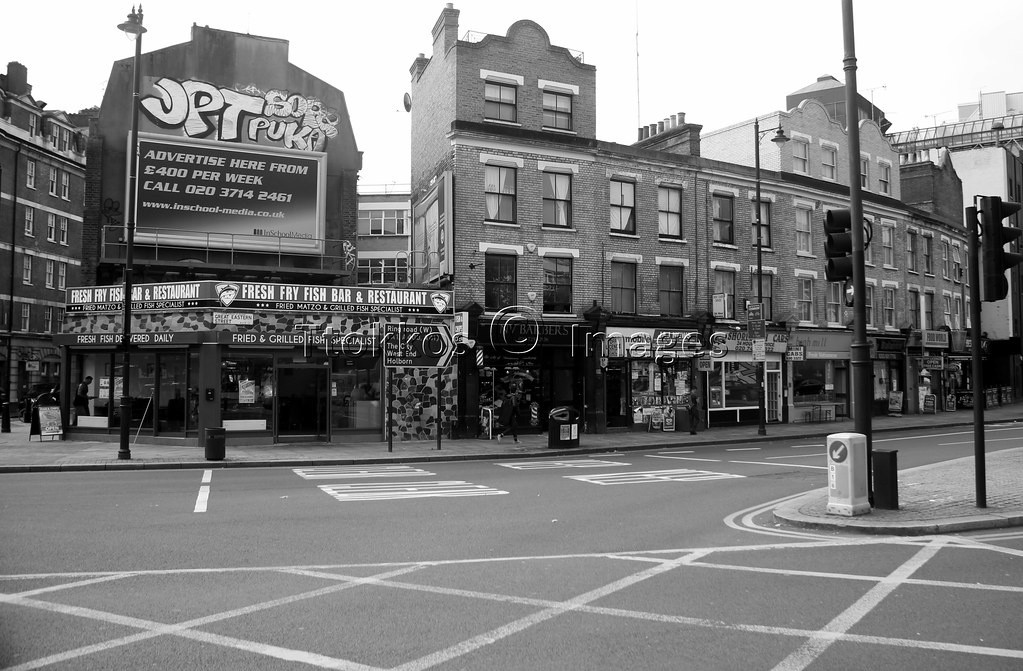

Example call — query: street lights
[871,85,887,121]
[117,1,148,457]
[753,116,792,435]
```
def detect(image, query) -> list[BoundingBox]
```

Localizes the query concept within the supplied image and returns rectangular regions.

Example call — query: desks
[812,402,845,424]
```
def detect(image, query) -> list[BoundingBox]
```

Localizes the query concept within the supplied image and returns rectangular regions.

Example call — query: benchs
[804,410,833,423]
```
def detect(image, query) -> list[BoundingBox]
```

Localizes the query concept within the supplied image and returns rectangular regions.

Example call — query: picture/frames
[105,362,123,377]
[146,363,166,378]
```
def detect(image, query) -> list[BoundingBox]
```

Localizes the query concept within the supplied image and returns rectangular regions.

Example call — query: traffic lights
[826,206,851,278]
[823,215,849,282]
[982,196,1023,305]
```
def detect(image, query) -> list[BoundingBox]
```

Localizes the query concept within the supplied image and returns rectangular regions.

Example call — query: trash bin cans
[547,407,581,449]
[870,448,899,510]
[204,426,226,461]
